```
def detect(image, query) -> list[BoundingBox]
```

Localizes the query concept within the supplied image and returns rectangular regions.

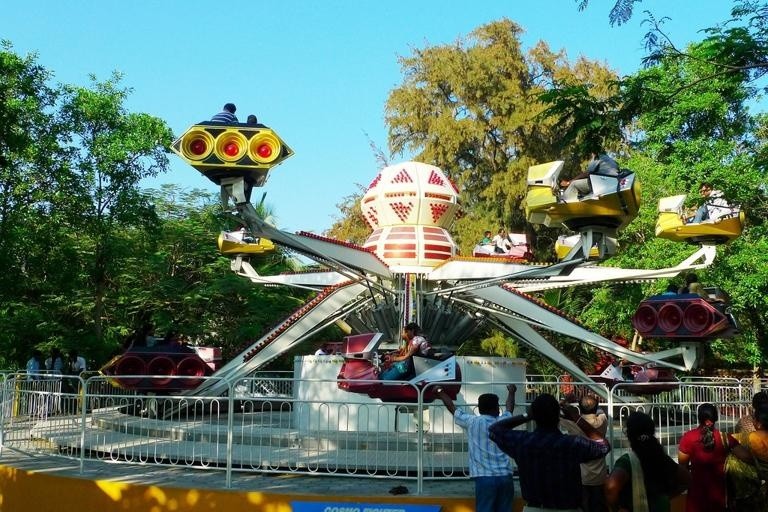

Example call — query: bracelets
[393,357,396,362]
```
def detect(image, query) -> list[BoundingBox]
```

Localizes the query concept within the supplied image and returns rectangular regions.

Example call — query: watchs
[567,180,570,186]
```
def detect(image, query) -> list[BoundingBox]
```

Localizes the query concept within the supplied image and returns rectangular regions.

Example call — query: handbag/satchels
[721,431,760,501]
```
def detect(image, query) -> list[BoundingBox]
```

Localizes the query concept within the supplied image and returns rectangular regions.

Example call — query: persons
[241,227,258,244]
[681,181,733,226]
[161,333,177,345]
[677,272,727,302]
[210,103,241,124]
[492,228,512,253]
[314,342,328,355]
[26,347,89,416]
[557,144,621,202]
[378,321,433,380]
[663,283,677,295]
[246,114,258,124]
[126,323,159,351]
[327,349,335,355]
[483,230,506,254]
[633,365,651,382]
[381,325,413,370]
